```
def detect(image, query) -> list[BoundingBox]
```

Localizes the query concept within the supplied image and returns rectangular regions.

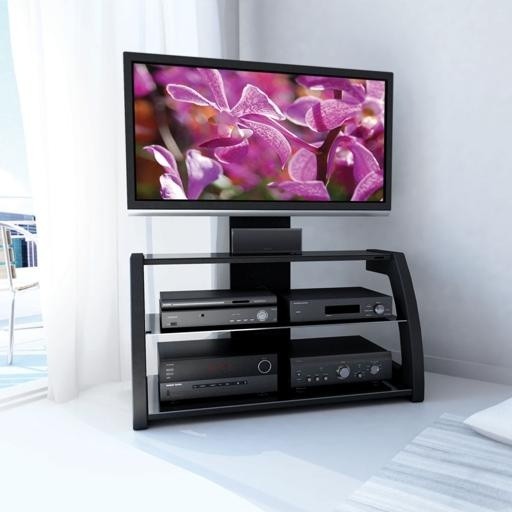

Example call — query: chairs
[0,221,40,364]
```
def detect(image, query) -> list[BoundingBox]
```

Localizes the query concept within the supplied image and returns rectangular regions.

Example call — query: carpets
[332,410,512,512]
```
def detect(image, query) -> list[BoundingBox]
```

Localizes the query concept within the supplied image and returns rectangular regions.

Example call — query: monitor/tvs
[122,50,394,228]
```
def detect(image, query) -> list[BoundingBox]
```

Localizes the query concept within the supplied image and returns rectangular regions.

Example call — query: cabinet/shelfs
[129,248,425,431]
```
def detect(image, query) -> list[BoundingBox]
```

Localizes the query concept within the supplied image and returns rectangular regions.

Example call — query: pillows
[462,397,512,446]
